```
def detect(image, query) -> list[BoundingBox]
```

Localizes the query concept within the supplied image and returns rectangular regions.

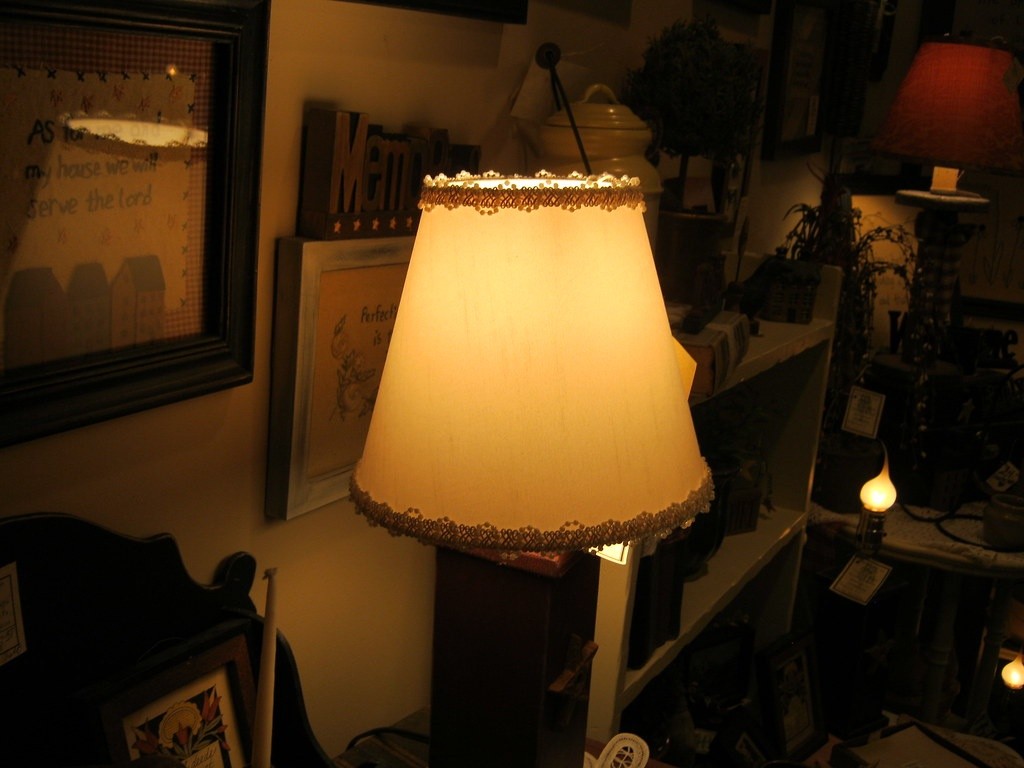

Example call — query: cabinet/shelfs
[427,259,848,768]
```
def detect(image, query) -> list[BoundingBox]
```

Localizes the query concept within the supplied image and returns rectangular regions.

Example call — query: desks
[808,491,1023,735]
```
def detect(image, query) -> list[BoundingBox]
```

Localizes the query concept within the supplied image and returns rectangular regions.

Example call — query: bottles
[982,493,1024,550]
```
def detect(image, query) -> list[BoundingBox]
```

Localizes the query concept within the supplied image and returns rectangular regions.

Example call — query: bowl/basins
[538,83,651,165]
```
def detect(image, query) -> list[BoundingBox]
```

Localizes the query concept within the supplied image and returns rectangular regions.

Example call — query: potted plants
[621,17,773,299]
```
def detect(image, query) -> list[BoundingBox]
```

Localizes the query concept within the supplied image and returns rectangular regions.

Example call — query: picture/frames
[0,1,271,444]
[755,629,829,764]
[78,618,260,768]
[269,234,417,520]
[761,0,843,154]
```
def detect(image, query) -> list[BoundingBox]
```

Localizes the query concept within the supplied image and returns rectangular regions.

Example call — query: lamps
[987,650,1024,733]
[855,440,898,559]
[871,40,1024,373]
[350,169,713,768]
